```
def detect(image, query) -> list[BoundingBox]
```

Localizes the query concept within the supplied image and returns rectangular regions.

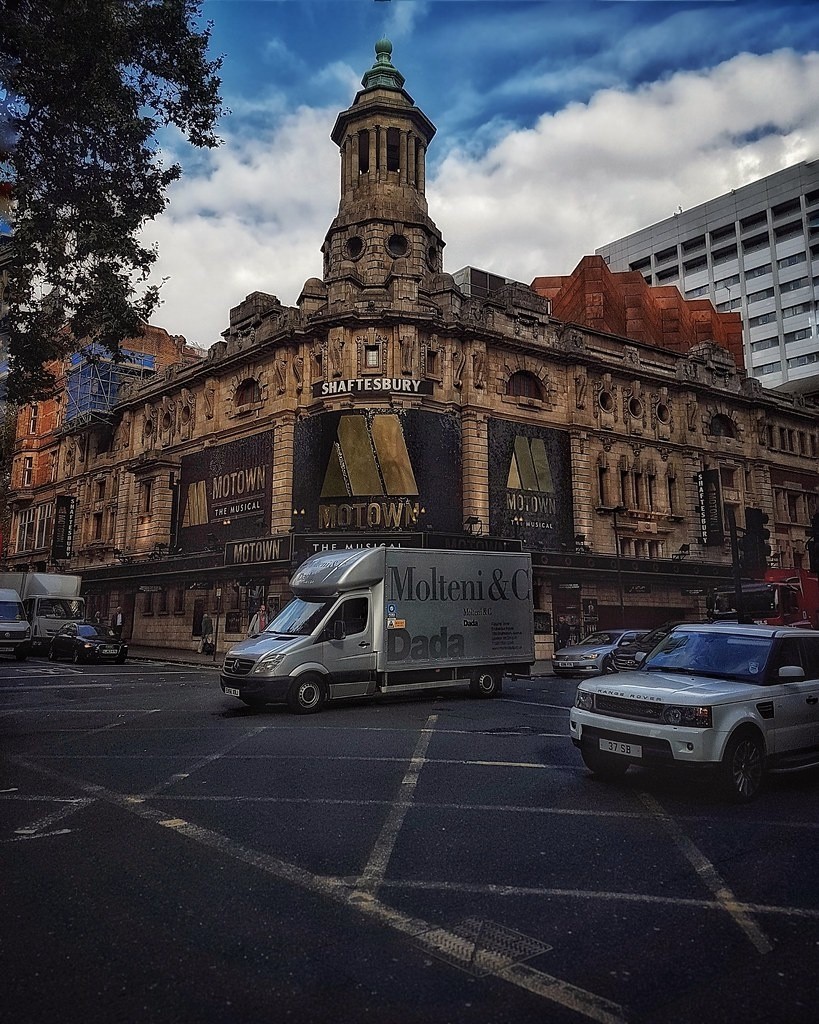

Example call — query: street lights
[596,505,632,630]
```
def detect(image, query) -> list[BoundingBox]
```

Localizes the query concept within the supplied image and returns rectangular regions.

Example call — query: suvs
[568,620,819,804]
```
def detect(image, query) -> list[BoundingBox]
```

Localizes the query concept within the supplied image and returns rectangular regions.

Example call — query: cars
[605,620,704,675]
[47,620,128,662]
[550,627,653,678]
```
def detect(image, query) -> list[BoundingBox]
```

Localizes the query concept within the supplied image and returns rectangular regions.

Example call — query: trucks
[0,571,85,656]
[706,568,819,631]
[220,547,541,712]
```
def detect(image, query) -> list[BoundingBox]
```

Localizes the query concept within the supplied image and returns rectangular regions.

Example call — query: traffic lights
[745,507,772,576]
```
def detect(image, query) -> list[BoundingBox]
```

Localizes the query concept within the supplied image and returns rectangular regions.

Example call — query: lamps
[22,502,733,579]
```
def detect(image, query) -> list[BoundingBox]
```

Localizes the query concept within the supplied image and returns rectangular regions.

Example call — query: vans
[0,588,32,660]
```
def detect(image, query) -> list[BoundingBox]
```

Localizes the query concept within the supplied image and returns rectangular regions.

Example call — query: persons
[196,614,213,654]
[91,611,103,625]
[555,615,570,651]
[737,650,775,682]
[110,606,126,638]
[247,604,269,637]
